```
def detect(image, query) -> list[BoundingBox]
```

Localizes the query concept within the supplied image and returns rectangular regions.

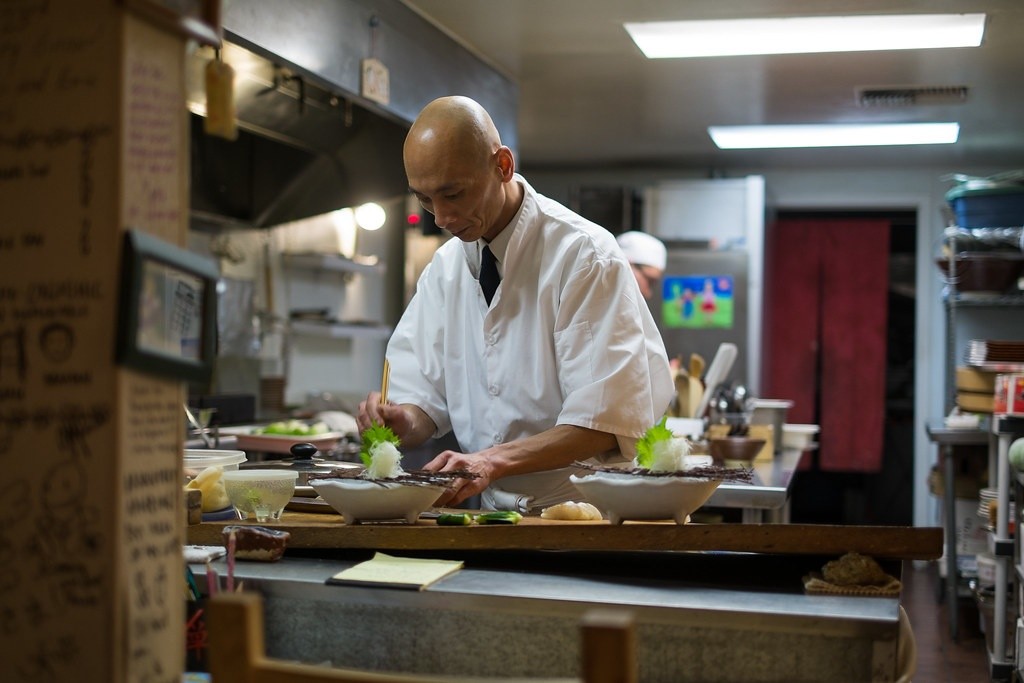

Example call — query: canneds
[992,372,1024,414]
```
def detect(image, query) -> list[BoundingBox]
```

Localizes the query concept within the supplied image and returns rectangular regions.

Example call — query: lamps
[201,44,238,141]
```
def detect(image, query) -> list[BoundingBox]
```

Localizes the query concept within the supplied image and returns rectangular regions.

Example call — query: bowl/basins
[568,473,722,525]
[709,438,767,467]
[183,449,247,511]
[781,424,819,449]
[222,470,299,522]
[936,257,1024,292]
[309,479,452,523]
[947,187,1023,230]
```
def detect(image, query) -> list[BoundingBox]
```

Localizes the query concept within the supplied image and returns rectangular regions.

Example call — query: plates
[976,487,1016,523]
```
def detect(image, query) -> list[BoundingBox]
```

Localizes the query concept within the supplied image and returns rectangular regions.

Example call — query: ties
[479,245,501,308]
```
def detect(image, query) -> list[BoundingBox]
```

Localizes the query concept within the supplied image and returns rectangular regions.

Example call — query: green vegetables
[359,417,401,467]
[635,414,672,466]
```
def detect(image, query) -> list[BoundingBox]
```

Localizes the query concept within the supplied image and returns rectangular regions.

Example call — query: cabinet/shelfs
[924,233,1023,683]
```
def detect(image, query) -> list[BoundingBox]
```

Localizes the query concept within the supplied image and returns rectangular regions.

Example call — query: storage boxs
[944,183,1024,229]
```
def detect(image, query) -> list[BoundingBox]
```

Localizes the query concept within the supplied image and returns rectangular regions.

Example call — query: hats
[616,231,668,270]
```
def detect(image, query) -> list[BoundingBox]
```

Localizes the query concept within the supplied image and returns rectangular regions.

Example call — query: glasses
[635,264,659,289]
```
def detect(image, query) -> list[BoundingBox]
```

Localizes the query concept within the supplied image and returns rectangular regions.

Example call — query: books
[324,552,465,592]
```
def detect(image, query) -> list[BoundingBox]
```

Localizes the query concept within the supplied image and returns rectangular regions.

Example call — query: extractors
[186,0,520,234]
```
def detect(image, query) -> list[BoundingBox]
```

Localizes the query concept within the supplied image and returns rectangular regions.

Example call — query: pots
[967,579,1017,658]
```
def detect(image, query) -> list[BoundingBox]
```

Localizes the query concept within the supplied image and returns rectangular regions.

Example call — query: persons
[616,230,679,382]
[355,95,676,513]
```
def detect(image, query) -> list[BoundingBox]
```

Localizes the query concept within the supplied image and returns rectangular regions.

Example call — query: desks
[696,433,805,524]
